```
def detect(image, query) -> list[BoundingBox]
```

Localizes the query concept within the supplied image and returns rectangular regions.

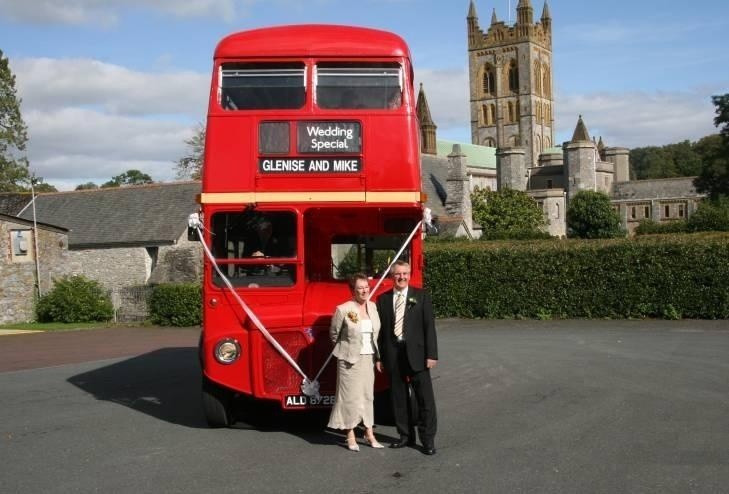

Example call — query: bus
[187,24,440,427]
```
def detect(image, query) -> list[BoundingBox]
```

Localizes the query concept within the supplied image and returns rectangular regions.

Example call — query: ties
[394,293,406,337]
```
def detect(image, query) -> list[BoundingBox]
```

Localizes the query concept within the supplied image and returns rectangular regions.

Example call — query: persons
[375,260,439,455]
[325,272,387,449]
[237,215,291,275]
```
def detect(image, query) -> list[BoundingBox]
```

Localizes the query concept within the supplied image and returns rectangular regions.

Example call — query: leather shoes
[362,434,385,449]
[390,437,436,455]
[344,436,360,453]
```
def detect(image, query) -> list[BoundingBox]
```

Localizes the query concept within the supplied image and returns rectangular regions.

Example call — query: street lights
[30,177,44,299]
